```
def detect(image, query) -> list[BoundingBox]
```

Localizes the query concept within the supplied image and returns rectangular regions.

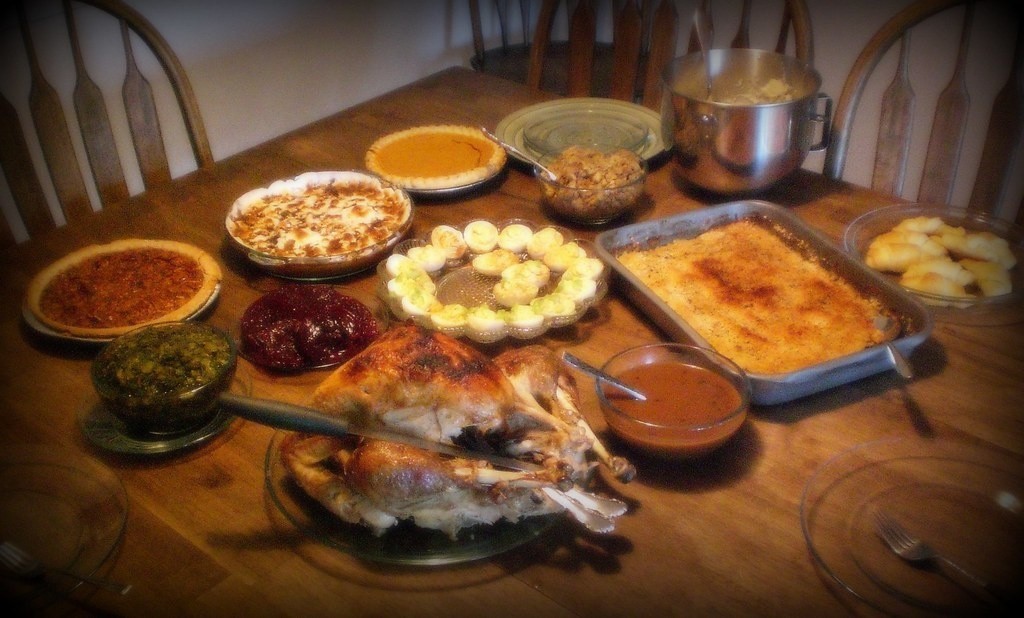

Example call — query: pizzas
[227,171,413,261]
[366,125,507,190]
[29,239,222,337]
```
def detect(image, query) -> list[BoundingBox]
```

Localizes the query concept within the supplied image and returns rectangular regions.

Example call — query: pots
[659,48,833,198]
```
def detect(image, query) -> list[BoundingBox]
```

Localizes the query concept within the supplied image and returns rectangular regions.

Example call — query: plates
[264,429,593,564]
[404,165,504,196]
[844,203,1024,309]
[495,98,665,165]
[20,282,222,342]
[225,169,416,281]
[76,377,246,455]
[373,219,612,344]
[227,285,389,368]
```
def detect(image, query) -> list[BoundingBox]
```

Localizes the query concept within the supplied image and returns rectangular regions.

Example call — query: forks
[870,510,1007,601]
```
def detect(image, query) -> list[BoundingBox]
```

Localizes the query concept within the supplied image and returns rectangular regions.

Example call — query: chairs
[1,0,214,252]
[469,0,815,114]
[824,1,1024,226]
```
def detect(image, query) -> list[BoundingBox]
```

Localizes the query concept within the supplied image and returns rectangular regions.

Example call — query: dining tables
[0,66,1024,618]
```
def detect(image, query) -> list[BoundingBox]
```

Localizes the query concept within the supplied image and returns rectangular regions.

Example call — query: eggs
[386,220,604,331]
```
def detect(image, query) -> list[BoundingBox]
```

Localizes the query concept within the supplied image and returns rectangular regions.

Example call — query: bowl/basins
[800,437,1024,618]
[90,320,237,433]
[594,343,752,462]
[533,144,649,224]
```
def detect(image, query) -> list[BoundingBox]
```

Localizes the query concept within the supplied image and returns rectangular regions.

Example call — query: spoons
[874,315,915,377]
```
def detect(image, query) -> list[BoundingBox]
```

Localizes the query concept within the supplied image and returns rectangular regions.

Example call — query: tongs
[218,392,628,533]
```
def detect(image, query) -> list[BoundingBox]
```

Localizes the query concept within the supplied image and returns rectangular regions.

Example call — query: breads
[864,214,1016,307]
